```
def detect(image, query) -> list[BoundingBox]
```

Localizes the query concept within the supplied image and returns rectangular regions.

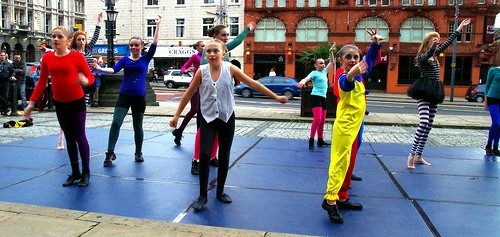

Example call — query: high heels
[172,129,182,147]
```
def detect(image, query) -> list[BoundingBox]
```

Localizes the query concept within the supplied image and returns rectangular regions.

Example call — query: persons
[483,66,500,157]
[39,42,55,112]
[38,11,104,151]
[320,24,383,225]
[190,21,257,177]
[147,65,179,85]
[170,40,205,147]
[92,55,106,101]
[97,56,107,69]
[88,13,162,168]
[23,25,96,187]
[295,40,338,151]
[168,37,290,211]
[12,53,28,110]
[269,67,276,76]
[406,17,473,172]
[0,49,15,103]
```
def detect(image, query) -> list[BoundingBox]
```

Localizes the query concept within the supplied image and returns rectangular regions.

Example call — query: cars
[18,62,47,104]
[233,76,301,100]
[163,70,194,88]
[465,84,486,103]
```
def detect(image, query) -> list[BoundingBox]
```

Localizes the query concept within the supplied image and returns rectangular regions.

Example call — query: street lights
[106,6,119,68]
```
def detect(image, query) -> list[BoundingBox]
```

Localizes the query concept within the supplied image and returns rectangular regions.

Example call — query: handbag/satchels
[2,117,34,127]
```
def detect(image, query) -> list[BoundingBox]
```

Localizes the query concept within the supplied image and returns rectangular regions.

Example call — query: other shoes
[407,155,416,169]
[494,146,500,156]
[485,145,493,155]
[308,138,315,151]
[350,173,361,181]
[413,157,431,166]
[317,139,332,147]
[0,102,12,116]
[90,102,98,107]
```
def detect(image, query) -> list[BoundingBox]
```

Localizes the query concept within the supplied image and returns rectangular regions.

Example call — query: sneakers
[57,142,64,150]
[135,152,144,162]
[209,157,219,167]
[102,151,116,167]
[193,195,207,210]
[321,199,343,222]
[62,173,81,186]
[78,170,90,187]
[216,192,232,203]
[336,196,362,210]
[191,159,200,175]
